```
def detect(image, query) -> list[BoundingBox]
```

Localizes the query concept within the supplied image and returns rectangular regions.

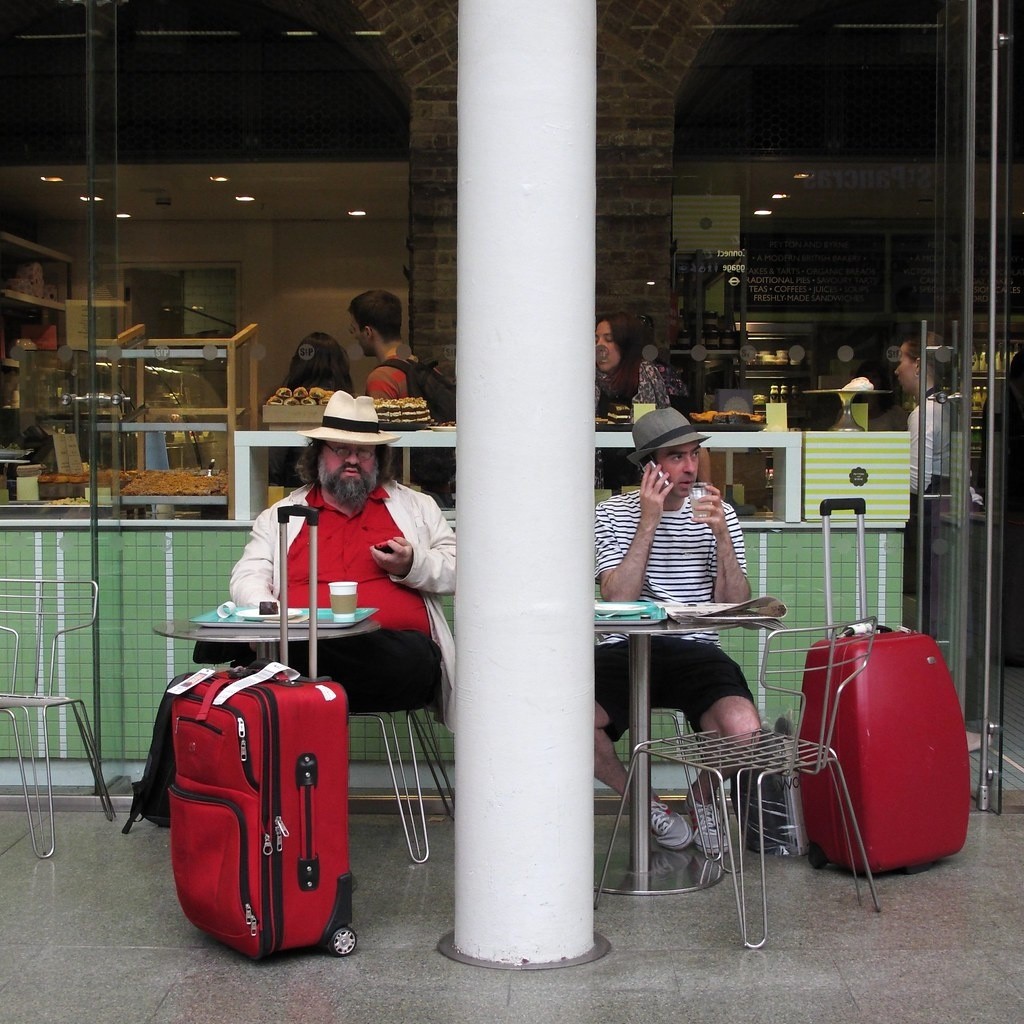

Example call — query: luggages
[792,496,972,875]
[161,504,359,963]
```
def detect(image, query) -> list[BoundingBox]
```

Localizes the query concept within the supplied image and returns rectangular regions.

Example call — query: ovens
[734,323,814,376]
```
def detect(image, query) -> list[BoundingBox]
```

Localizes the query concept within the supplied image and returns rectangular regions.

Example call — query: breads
[265,387,335,405]
[36,472,86,483]
[596,404,632,425]
[689,410,766,424]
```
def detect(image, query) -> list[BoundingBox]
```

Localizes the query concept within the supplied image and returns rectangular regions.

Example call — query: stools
[348,704,459,862]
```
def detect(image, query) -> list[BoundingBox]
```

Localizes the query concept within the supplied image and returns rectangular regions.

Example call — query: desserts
[371,397,431,423]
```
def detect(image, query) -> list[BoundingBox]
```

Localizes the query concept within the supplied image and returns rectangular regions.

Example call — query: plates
[596,603,649,615]
[378,422,427,432]
[428,426,456,431]
[236,608,301,621]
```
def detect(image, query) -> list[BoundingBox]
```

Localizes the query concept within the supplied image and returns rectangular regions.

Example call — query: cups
[689,482,714,518]
[329,582,358,619]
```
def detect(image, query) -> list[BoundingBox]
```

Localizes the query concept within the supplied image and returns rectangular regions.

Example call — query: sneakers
[682,784,730,855]
[649,788,694,850]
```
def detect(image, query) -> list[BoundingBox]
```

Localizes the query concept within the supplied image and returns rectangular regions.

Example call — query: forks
[596,611,618,618]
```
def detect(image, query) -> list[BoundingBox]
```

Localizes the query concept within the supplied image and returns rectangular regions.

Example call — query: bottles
[996,342,1019,371]
[766,469,773,512]
[790,386,802,431]
[706,330,720,350]
[780,385,788,403]
[722,330,734,350]
[972,344,988,370]
[701,330,705,348]
[678,330,692,349]
[704,310,719,329]
[972,386,988,412]
[770,386,779,402]
[971,425,982,451]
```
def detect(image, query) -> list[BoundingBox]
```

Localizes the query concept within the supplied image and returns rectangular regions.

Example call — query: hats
[625,407,711,467]
[298,389,403,447]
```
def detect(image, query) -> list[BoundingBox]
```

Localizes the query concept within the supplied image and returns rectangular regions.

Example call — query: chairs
[592,617,880,949]
[0,578,118,856]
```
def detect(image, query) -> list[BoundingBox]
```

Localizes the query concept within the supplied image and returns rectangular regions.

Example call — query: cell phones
[374,539,395,554]
[642,461,669,489]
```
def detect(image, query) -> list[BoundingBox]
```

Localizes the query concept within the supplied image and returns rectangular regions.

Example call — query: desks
[156,620,380,673]
[595,623,743,896]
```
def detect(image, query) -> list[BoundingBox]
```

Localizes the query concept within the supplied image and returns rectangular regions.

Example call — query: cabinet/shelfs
[1,231,260,521]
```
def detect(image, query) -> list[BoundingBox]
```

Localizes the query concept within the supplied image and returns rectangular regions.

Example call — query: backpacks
[366,356,457,496]
[120,670,198,834]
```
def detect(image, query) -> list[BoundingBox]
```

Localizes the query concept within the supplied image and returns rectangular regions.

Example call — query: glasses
[347,324,374,335]
[322,441,377,462]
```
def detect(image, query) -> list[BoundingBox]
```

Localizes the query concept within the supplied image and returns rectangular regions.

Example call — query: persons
[347,290,454,508]
[893,331,951,633]
[228,389,456,730]
[267,331,353,487]
[595,314,712,490]
[853,360,907,432]
[977,349,1024,512]
[593,407,761,853]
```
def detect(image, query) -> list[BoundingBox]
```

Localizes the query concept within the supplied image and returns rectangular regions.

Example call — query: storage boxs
[261,405,328,431]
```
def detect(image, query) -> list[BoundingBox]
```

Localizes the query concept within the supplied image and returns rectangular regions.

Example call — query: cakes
[8,338,37,350]
